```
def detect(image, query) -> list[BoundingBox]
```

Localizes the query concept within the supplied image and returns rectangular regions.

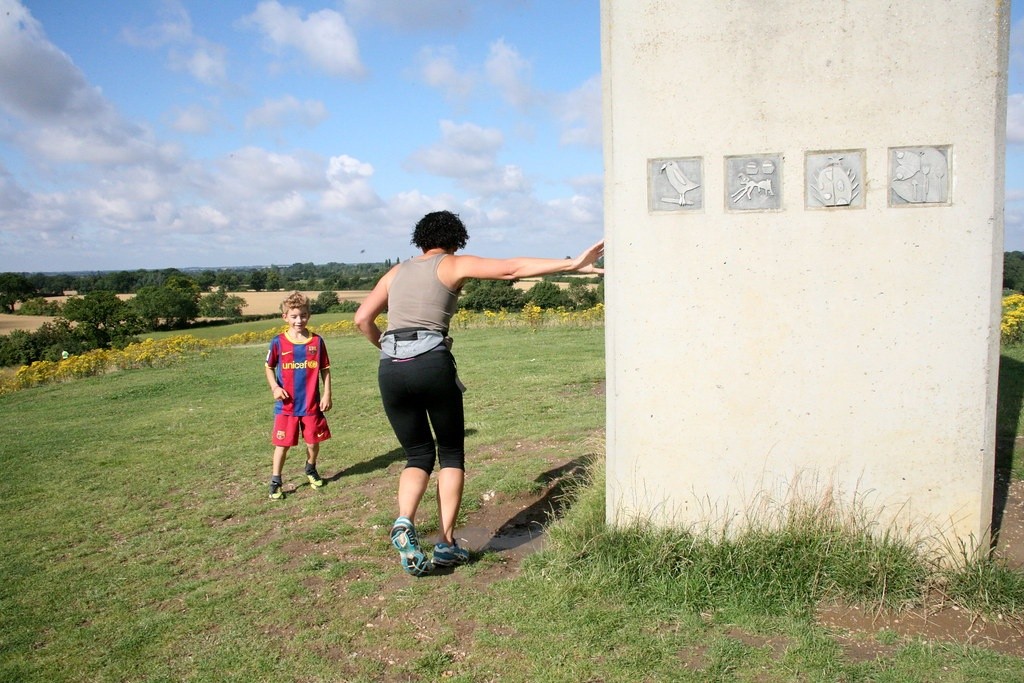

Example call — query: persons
[350,208,606,576]
[265,293,333,502]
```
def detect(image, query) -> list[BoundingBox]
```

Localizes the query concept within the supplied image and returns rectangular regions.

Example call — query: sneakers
[430,538,469,568]
[269,480,284,502]
[389,516,434,576]
[305,466,324,490]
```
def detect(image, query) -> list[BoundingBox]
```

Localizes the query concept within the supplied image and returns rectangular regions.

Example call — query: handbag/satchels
[379,327,445,359]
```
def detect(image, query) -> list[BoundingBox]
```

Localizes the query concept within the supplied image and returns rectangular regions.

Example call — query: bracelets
[272,385,280,393]
